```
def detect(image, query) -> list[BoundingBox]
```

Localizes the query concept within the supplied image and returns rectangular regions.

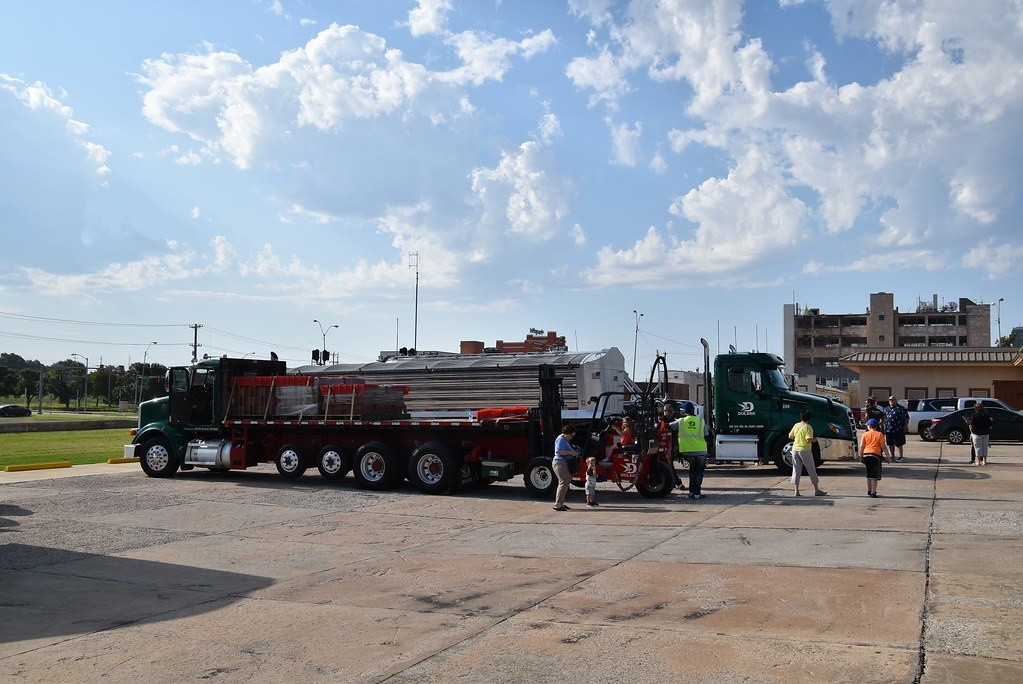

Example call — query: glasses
[571,433,574,437]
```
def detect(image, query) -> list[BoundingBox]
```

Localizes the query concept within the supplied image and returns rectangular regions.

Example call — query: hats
[663,404,675,411]
[683,402,693,413]
[888,396,895,400]
[866,419,878,425]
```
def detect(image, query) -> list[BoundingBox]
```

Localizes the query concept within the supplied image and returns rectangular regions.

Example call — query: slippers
[553,504,571,511]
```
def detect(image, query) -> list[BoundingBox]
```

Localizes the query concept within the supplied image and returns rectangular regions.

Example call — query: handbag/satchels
[564,456,581,475]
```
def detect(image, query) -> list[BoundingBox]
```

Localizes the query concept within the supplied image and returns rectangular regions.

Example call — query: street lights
[139,342,157,405]
[633,310,644,383]
[991,298,1004,347]
[314,320,339,350]
[71,354,88,411]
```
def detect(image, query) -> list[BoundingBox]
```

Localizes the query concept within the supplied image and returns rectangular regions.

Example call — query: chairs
[622,430,642,452]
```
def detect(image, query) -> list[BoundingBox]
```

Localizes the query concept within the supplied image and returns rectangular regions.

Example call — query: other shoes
[969,461,986,465]
[587,500,599,507]
[689,493,705,499]
[815,489,827,496]
[896,457,905,463]
[887,457,897,463]
[794,490,800,496]
[867,490,877,497]
[673,483,685,490]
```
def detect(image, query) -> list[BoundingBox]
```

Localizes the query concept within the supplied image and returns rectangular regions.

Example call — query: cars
[917,398,977,412]
[929,407,1023,445]
[0,404,31,417]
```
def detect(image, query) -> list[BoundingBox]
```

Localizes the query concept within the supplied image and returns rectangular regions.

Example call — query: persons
[552,423,579,512]
[858,418,892,498]
[662,400,711,499]
[964,402,992,466]
[788,409,827,496]
[601,415,634,462]
[585,456,599,508]
[864,394,910,463]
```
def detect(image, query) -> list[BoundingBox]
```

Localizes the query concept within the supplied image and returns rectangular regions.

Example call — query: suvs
[523,391,676,500]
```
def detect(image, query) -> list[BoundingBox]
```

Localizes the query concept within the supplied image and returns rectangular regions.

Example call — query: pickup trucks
[908,397,1023,442]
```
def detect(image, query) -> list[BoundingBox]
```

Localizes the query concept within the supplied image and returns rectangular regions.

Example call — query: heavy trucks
[123,351,566,493]
[622,338,859,476]
